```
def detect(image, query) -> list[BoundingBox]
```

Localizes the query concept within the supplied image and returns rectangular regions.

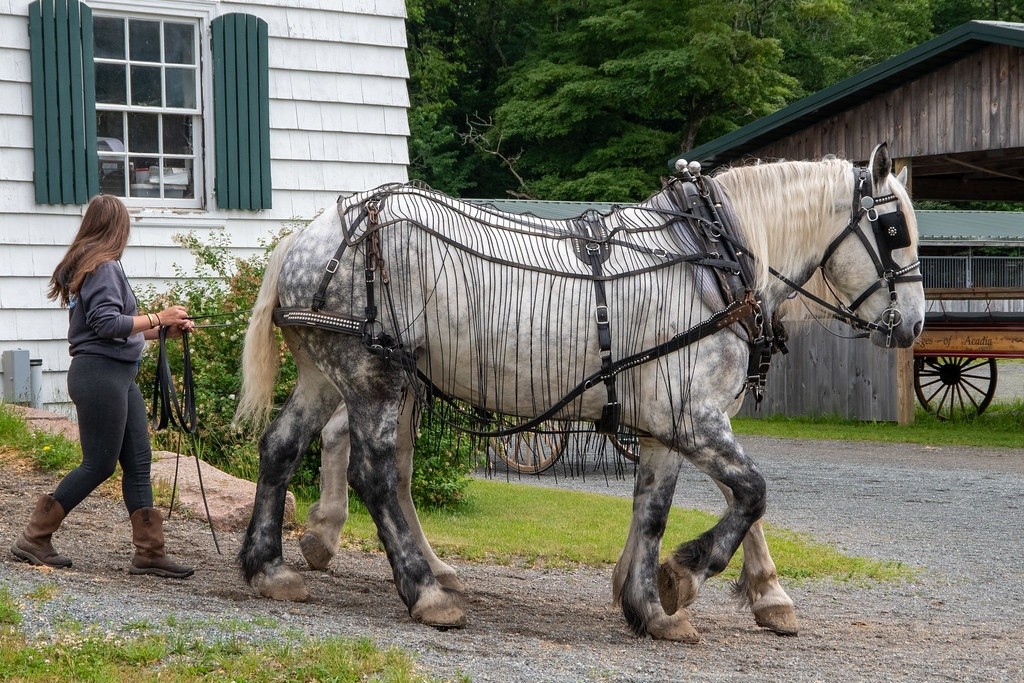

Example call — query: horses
[230,139,926,645]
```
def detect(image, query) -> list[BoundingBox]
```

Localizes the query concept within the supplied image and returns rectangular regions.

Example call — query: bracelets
[147,313,154,329]
[154,312,160,326]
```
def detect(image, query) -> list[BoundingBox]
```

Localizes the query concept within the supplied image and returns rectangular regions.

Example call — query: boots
[128,508,195,577]
[10,493,73,571]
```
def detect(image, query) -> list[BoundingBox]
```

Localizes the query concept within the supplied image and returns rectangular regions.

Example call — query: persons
[11,196,194,579]
